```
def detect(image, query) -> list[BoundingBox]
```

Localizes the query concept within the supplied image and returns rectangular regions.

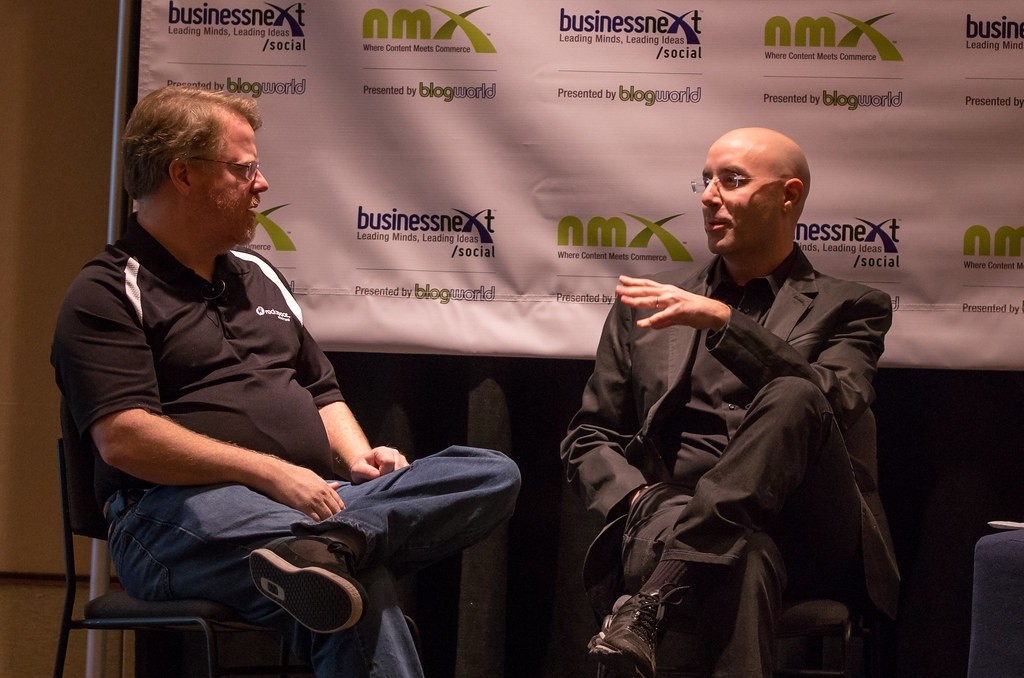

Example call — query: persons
[51,84,522,678]
[560,128,900,678]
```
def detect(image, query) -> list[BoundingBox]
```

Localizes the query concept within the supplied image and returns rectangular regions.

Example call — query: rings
[656,296,659,308]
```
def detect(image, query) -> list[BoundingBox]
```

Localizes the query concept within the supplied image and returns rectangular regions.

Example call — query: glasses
[186,156,260,182]
[690,173,787,193]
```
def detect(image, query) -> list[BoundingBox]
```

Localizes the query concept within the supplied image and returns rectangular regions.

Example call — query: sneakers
[587,583,690,678]
[249,536,368,633]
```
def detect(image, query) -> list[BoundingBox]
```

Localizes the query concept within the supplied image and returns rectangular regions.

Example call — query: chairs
[53,393,295,677]
